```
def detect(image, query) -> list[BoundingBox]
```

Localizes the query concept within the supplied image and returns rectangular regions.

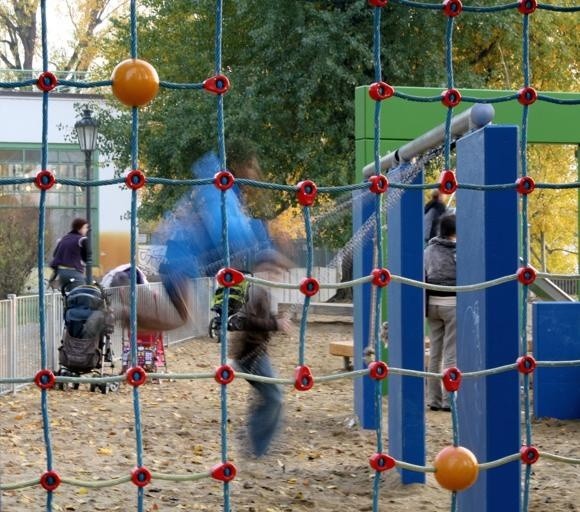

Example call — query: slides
[529,263,574,303]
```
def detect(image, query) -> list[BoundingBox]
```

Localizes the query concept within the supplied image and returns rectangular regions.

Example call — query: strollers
[54,277,120,394]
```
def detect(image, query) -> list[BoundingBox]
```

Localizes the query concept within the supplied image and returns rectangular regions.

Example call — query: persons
[115,139,299,338]
[424,188,448,247]
[49,218,94,290]
[424,215,456,411]
[99,261,148,288]
[225,249,295,463]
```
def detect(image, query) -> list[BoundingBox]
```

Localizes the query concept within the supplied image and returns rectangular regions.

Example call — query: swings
[153,145,446,388]
